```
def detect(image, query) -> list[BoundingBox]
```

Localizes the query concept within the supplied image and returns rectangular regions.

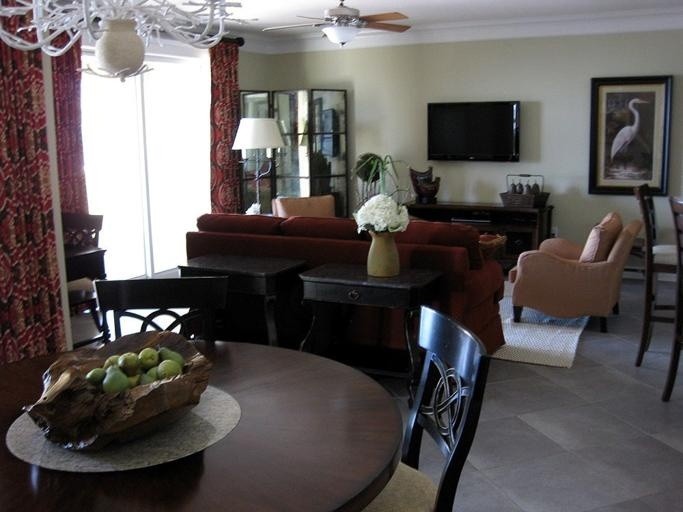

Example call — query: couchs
[191,211,507,395]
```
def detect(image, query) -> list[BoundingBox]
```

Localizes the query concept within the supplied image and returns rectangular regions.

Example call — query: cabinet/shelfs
[403,200,553,272]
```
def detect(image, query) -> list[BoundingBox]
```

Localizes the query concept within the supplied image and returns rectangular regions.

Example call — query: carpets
[490,269,596,369]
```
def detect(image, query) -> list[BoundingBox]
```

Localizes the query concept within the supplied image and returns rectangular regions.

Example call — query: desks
[174,250,455,411]
[1,337,406,511]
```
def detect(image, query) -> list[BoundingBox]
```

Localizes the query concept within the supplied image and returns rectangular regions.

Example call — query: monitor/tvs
[427,100,521,162]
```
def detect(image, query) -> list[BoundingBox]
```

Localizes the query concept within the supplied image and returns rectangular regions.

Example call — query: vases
[368,224,403,280]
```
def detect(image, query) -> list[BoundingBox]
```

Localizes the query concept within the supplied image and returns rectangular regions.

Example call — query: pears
[86,347,185,394]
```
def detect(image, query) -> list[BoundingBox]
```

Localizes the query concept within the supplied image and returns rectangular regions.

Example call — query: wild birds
[610,98,649,170]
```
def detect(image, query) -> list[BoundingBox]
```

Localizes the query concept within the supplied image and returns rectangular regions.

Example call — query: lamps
[1,0,248,87]
[229,115,286,214]
[319,26,363,46]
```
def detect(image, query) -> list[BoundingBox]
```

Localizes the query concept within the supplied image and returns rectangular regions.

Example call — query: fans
[260,1,414,48]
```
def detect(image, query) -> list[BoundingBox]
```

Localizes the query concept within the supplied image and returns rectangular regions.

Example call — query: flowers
[353,189,412,237]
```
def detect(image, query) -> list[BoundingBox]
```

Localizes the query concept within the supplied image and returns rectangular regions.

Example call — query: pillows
[581,210,623,265]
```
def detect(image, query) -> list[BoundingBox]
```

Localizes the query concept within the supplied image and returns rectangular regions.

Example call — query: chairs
[513,218,642,334]
[622,181,683,405]
[273,194,340,218]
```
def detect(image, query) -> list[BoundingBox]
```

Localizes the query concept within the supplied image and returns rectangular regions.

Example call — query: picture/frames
[588,75,678,196]
